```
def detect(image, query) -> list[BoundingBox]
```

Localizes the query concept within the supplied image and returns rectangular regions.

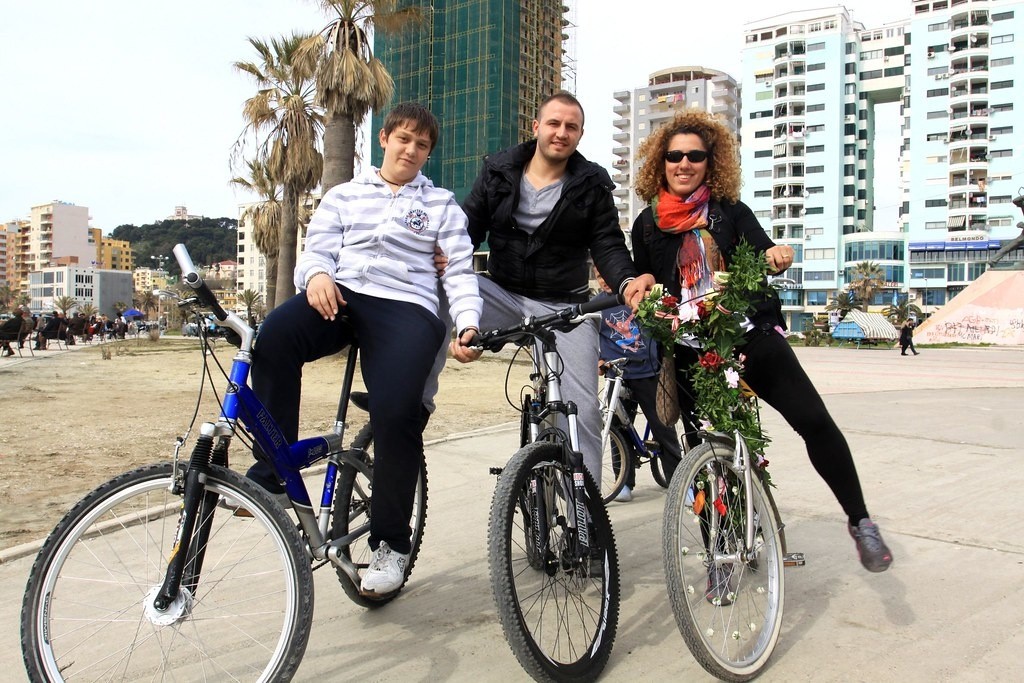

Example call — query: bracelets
[459,328,478,337]
[307,271,331,289]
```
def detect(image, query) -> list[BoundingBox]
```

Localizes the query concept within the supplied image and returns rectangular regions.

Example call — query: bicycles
[20,243,428,683]
[552,260,806,683]
[459,293,626,683]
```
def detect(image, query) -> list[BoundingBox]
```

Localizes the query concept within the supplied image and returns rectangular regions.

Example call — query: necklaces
[378,170,405,187]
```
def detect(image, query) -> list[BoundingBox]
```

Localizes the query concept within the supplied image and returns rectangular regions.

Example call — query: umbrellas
[122,309,144,316]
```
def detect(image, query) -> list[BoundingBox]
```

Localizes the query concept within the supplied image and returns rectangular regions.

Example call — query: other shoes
[914,352,920,355]
[352,389,431,431]
[580,525,603,578]
[901,353,908,355]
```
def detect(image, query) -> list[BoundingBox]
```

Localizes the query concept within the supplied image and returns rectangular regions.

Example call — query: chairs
[72,318,91,345]
[36,317,70,351]
[0,318,35,358]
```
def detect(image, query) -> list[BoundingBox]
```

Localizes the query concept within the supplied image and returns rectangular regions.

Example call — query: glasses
[665,149,711,163]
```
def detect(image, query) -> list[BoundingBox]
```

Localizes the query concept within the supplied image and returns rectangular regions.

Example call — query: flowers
[636,233,779,536]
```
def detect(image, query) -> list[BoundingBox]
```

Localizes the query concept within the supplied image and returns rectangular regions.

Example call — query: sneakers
[847,516,892,573]
[614,484,632,502]
[225,488,292,510]
[684,487,696,508]
[361,538,411,597]
[701,559,733,605]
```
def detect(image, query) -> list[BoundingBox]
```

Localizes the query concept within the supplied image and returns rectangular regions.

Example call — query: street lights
[908,273,913,318]
[925,278,928,320]
[158,290,173,334]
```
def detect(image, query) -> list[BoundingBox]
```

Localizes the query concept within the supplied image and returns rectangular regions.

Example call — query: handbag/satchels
[657,360,680,428]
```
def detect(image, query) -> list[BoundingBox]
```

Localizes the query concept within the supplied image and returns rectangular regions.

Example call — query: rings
[782,255,789,258]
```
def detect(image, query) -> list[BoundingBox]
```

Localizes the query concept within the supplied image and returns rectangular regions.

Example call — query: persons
[348,91,655,577]
[0,305,33,356]
[630,112,891,604]
[243,103,485,599]
[900,318,919,356]
[589,261,695,506]
[31,311,128,350]
